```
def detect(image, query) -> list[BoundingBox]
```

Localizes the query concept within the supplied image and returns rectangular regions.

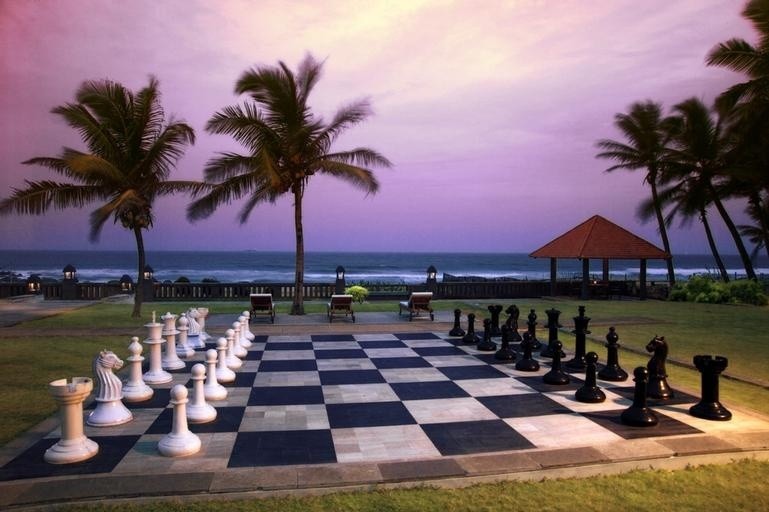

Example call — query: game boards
[1,327,768,506]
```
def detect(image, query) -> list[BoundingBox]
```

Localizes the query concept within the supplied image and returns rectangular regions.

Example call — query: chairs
[246,292,436,324]
